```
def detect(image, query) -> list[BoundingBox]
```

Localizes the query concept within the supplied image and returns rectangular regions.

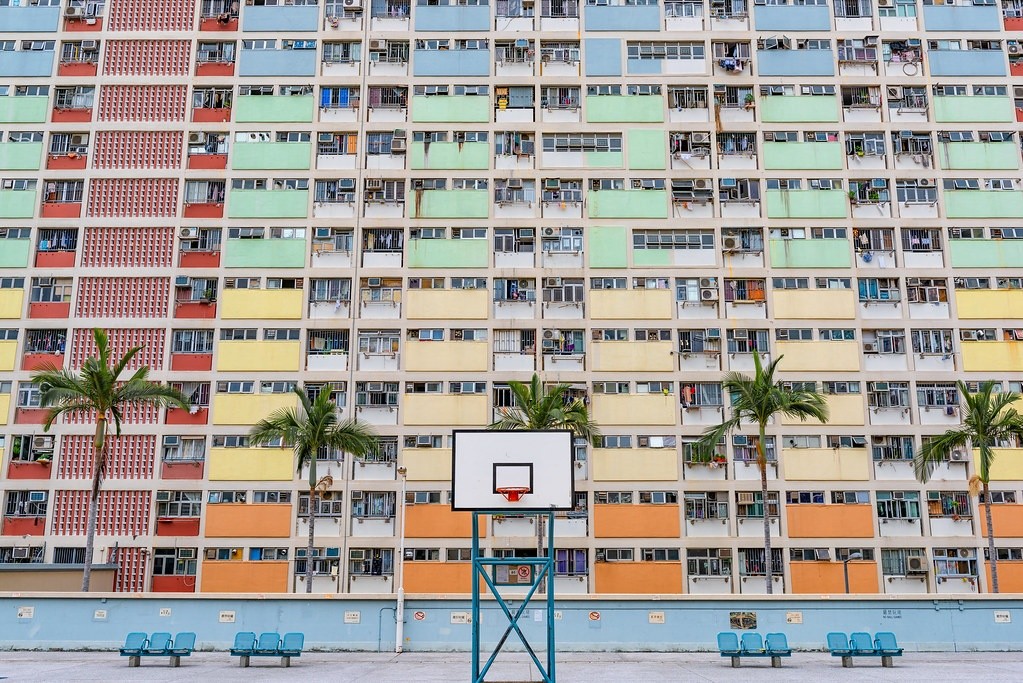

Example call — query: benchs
[826,630,903,668]
[229,631,306,669]
[716,631,793,668]
[120,632,197,669]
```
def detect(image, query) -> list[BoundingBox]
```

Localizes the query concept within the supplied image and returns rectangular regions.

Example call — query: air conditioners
[72,133,88,146]
[37,277,53,288]
[29,491,48,503]
[63,6,83,17]
[156,490,173,502]
[11,548,29,559]
[180,226,198,239]
[82,40,98,51]
[178,548,195,560]
[31,436,55,451]
[163,435,181,446]
[307,0,1021,595]
[188,131,207,145]
[175,276,192,287]
